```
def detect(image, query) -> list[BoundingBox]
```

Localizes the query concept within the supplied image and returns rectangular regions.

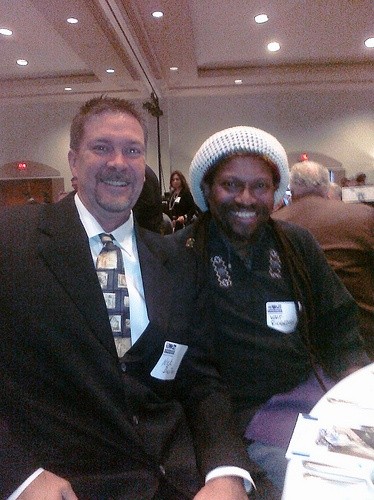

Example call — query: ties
[95,233,133,357]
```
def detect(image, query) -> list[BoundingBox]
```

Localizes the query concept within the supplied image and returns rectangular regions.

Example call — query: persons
[0,92,277,500]
[23,177,78,204]
[272,161,374,362]
[172,127,371,489]
[133,164,197,235]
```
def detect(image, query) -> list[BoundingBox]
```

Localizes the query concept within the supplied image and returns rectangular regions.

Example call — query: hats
[187,126,289,214]
